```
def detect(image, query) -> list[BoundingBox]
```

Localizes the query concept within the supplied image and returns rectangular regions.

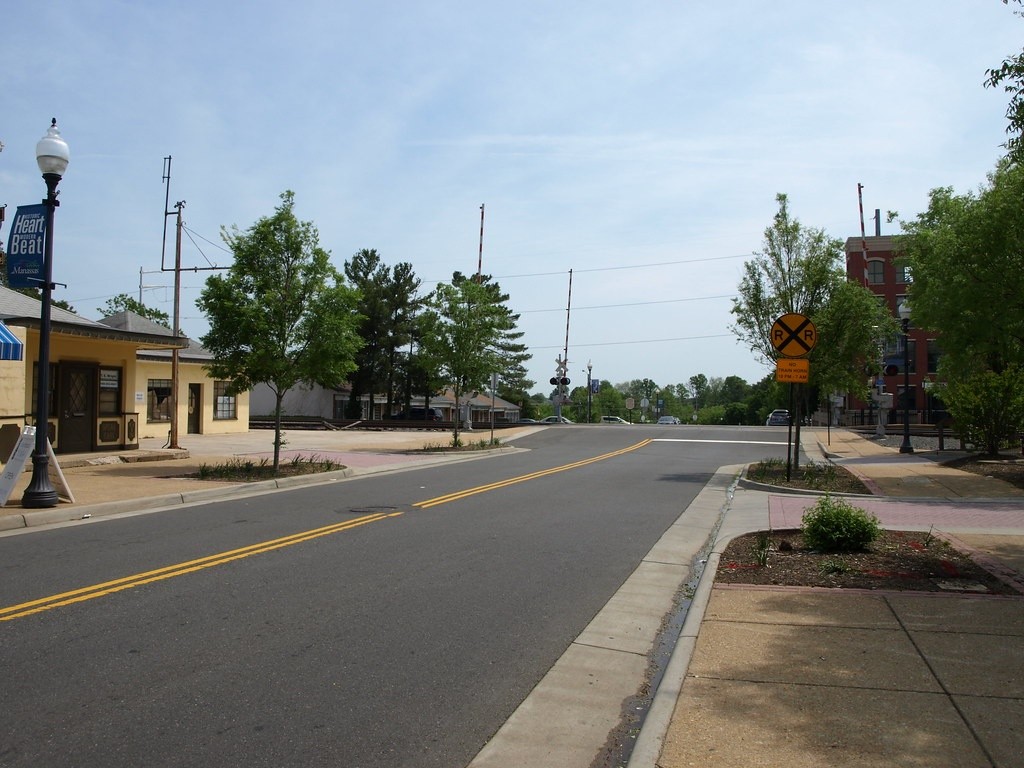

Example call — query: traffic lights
[883,364,899,375]
[560,378,570,385]
[550,377,559,385]
[865,363,879,376]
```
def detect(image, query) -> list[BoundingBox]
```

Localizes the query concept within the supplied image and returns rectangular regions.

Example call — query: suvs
[382,406,443,421]
[766,410,790,426]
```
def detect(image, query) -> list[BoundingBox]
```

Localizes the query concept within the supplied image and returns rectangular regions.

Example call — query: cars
[656,416,675,424]
[516,418,537,422]
[766,413,771,425]
[675,417,681,424]
[537,415,575,423]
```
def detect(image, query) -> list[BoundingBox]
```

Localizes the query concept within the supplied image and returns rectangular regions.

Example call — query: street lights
[21,116,70,507]
[896,297,914,454]
[587,359,591,424]
[656,389,659,422]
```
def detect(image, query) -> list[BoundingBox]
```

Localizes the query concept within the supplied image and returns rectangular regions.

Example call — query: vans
[600,416,634,424]
